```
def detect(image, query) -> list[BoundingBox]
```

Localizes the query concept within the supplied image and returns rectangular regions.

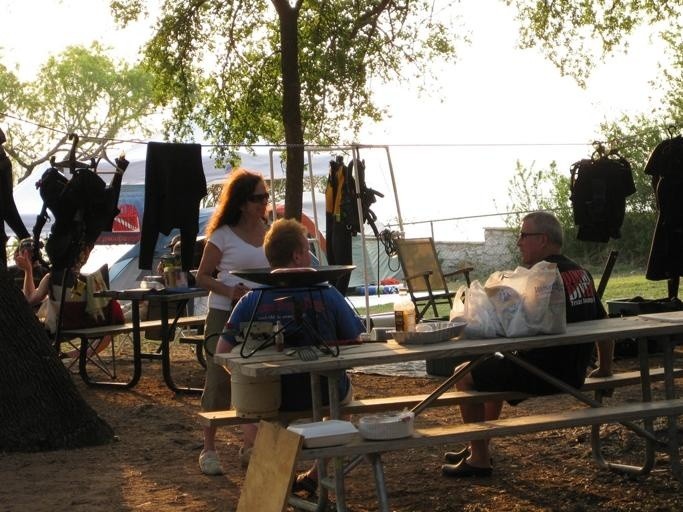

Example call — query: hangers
[84,139,128,176]
[570,125,683,174]
[47,133,97,172]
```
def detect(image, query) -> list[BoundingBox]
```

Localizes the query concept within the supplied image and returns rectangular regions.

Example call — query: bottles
[360,278,403,295]
[393,288,416,333]
[274,320,284,352]
[141,254,183,294]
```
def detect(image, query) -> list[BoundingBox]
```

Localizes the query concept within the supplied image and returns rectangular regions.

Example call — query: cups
[188,270,199,278]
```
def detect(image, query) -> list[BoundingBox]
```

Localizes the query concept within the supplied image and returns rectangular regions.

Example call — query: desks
[211,308,683,511]
[79,282,204,399]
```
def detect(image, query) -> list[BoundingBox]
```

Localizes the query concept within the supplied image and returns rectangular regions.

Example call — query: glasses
[521,232,544,238]
[247,192,269,203]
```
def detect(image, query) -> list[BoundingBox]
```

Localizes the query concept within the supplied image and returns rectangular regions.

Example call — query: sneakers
[238,445,253,464]
[198,447,224,476]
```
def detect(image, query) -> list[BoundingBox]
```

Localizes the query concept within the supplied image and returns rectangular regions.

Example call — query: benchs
[59,312,209,345]
[289,395,681,512]
[192,363,683,478]
[178,331,206,369]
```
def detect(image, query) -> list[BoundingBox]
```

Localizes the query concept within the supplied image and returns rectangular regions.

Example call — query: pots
[229,265,357,288]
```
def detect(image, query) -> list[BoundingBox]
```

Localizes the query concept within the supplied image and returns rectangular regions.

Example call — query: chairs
[390,235,478,325]
[44,263,133,383]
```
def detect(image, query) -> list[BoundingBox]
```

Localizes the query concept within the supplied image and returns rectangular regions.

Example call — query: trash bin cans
[605,297,683,357]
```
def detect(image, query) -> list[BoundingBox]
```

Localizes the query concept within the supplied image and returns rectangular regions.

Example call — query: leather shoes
[292,472,318,499]
[440,457,494,479]
[444,446,471,464]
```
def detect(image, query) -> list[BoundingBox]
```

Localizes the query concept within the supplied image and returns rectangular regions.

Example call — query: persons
[195,171,271,477]
[213,217,366,495]
[7,232,208,362]
[439,211,614,478]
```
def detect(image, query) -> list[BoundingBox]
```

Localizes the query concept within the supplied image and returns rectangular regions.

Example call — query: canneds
[164,267,188,288]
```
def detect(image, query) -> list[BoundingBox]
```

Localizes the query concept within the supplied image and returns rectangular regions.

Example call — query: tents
[108,204,215,290]
[107,205,409,297]
